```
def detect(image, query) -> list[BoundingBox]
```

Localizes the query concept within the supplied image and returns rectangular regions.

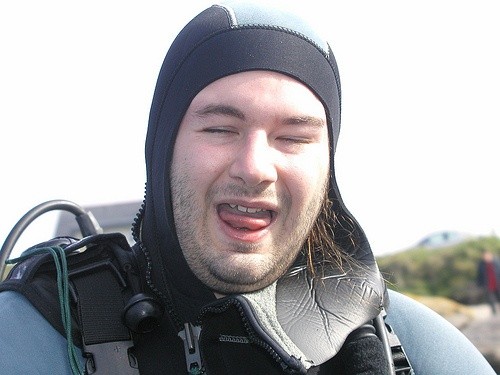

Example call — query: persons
[0,5,497,375]
